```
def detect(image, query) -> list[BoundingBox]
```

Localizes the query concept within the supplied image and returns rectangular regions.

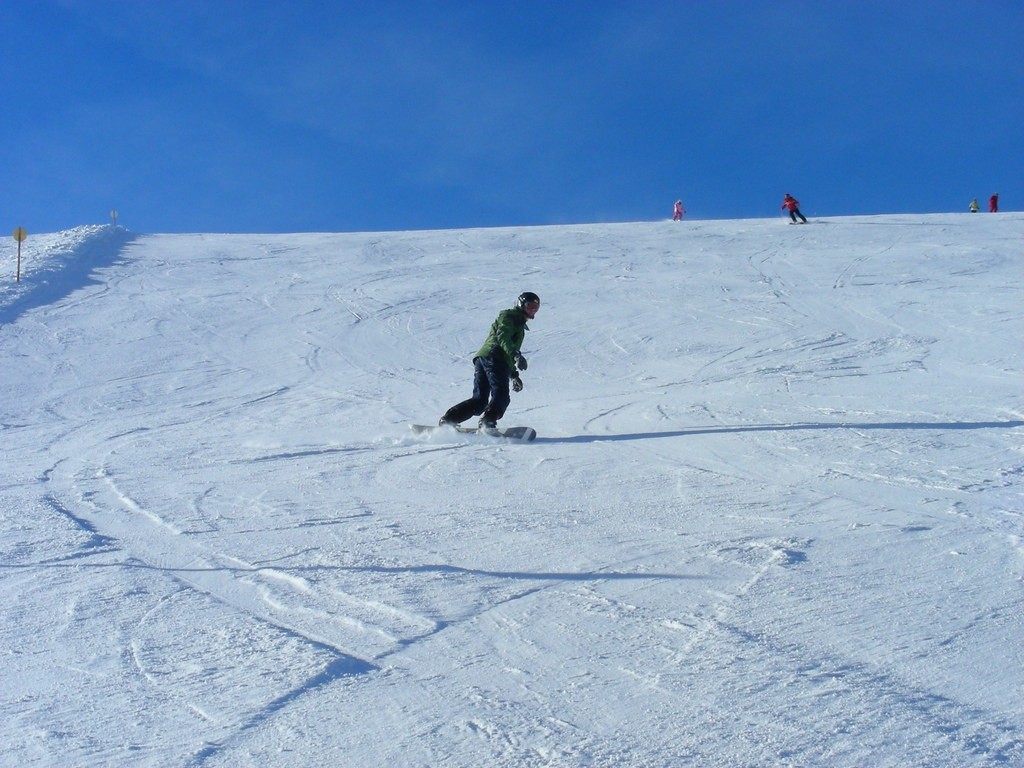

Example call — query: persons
[989,193,998,212]
[672,200,686,221]
[439,292,541,437]
[781,193,807,224]
[969,198,979,213]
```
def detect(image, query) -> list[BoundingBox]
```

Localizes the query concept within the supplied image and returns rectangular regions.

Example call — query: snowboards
[409,424,536,443]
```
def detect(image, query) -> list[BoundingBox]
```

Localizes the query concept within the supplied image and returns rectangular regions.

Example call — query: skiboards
[789,222,807,224]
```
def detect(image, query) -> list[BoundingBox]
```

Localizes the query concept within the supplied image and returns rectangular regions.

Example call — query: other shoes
[479,419,499,437]
[439,417,457,428]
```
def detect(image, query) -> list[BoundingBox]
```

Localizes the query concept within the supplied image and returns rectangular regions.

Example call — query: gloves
[512,377,523,392]
[514,354,527,370]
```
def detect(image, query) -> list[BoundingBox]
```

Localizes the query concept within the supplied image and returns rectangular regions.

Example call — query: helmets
[784,193,790,201]
[517,292,541,319]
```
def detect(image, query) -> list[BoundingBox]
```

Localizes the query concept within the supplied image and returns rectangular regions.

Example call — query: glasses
[524,301,539,312]
